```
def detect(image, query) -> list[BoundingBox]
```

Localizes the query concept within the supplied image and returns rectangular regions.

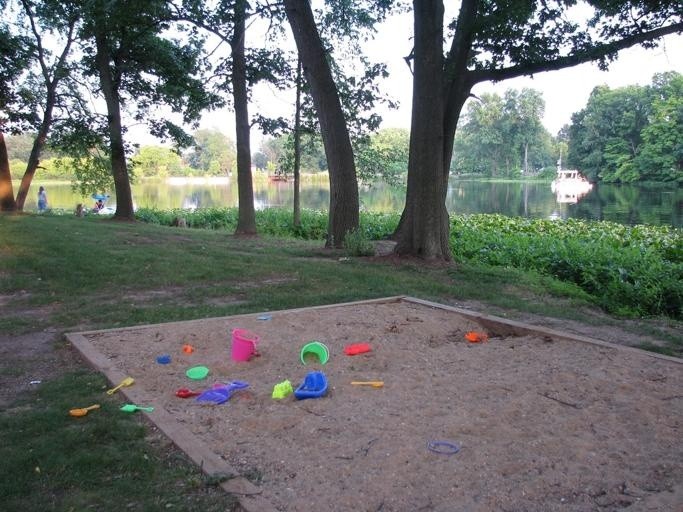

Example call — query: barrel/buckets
[301,341,329,365]
[231,328,259,362]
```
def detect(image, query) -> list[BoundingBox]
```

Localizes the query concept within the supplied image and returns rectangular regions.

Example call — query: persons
[36,186,47,217]
[75,203,87,218]
[92,200,104,214]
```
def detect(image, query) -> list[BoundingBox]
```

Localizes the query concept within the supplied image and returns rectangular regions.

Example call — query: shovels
[196,381,248,404]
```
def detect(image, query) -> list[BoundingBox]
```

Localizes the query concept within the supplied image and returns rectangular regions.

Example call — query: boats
[552,169,583,183]
[550,183,594,204]
[268,174,294,183]
